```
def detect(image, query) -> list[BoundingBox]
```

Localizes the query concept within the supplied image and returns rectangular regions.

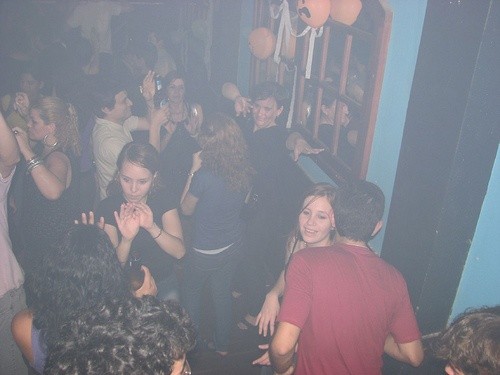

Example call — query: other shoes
[203,337,228,356]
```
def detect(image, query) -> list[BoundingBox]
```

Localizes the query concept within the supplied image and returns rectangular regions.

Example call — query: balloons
[247,0,363,59]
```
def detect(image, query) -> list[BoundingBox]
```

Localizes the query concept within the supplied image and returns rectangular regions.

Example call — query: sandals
[236,313,258,331]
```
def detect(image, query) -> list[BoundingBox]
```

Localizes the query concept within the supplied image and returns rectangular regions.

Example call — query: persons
[269,179,425,375]
[0,0,500,375]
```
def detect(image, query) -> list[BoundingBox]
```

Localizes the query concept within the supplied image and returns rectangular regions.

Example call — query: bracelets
[187,173,194,176]
[150,228,163,239]
[23,156,45,176]
